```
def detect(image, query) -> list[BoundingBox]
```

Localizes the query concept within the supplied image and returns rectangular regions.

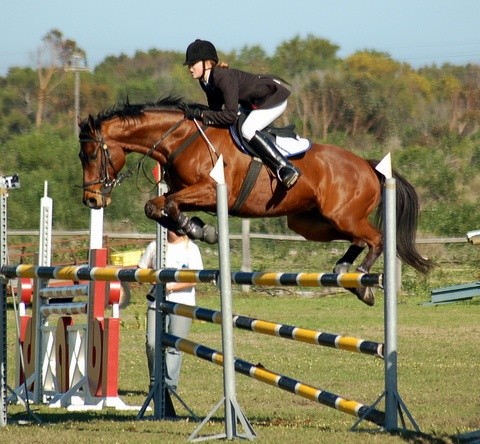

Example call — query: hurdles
[0,153,261,443]
[134,151,424,436]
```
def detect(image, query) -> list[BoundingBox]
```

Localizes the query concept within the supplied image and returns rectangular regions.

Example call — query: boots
[249,130,301,187]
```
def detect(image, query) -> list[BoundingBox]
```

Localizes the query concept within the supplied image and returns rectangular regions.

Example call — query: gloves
[185,108,201,119]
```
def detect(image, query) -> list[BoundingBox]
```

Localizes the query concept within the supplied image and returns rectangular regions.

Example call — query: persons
[136,227,206,396]
[183,39,302,182]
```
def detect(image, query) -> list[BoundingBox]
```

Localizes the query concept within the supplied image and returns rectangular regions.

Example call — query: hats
[183,39,218,65]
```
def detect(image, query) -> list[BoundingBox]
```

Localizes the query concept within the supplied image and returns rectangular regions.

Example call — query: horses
[78,95,433,307]
